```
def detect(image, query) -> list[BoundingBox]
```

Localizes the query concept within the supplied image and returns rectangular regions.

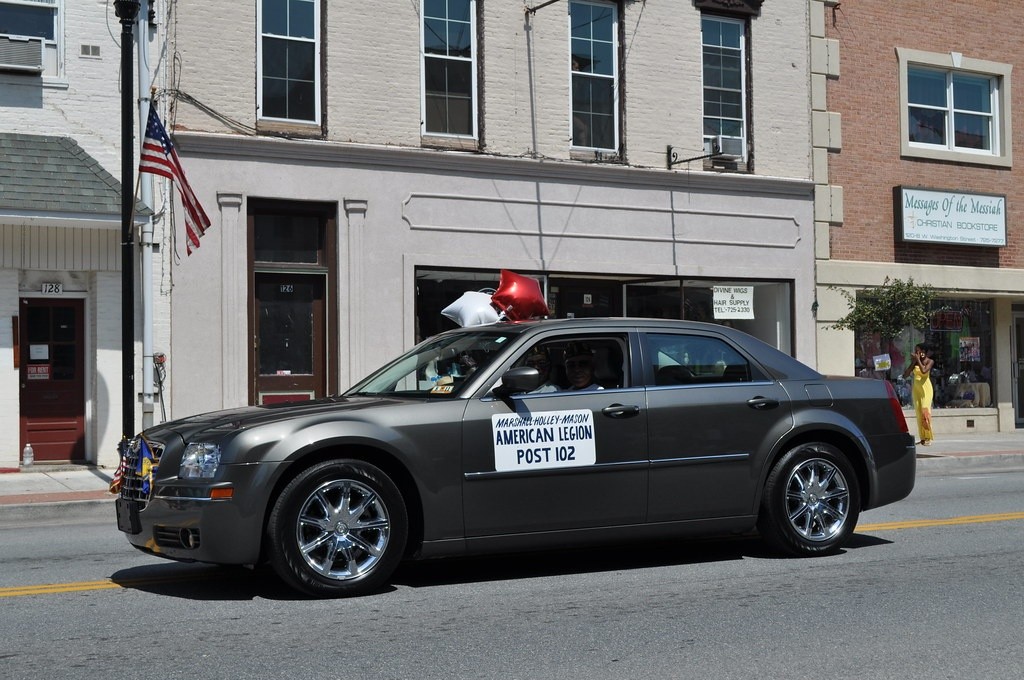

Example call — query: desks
[956,381,991,408]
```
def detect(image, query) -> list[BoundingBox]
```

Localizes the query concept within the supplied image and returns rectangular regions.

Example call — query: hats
[527,345,547,356]
[564,344,600,360]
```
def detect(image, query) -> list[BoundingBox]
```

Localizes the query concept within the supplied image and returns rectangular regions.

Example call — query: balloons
[491,268,551,321]
[441,290,503,328]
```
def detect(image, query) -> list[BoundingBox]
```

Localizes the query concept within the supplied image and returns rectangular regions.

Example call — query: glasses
[563,359,593,368]
[525,358,550,367]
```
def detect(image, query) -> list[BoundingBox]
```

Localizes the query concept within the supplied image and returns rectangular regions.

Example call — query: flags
[139,100,212,258]
[135,434,160,493]
[110,440,128,494]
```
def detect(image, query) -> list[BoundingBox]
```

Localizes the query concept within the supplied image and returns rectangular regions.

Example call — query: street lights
[112,0,142,468]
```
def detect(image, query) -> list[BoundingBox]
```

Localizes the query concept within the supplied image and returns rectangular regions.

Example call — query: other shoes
[922,440,931,446]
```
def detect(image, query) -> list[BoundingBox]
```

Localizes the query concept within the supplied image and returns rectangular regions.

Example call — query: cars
[106,316,918,598]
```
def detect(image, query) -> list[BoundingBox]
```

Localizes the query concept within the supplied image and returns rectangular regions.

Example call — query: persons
[516,346,560,394]
[558,341,605,391]
[902,343,935,447]
